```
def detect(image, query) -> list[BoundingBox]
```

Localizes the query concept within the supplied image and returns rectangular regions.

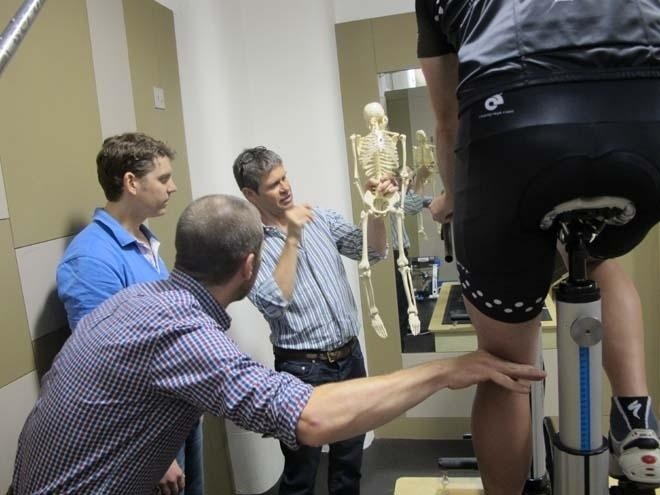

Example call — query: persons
[230,145,400,495]
[413,0,660,495]
[386,162,434,352]
[54,133,207,495]
[5,194,549,495]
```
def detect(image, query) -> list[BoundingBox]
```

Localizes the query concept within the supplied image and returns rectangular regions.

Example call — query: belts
[275,338,357,364]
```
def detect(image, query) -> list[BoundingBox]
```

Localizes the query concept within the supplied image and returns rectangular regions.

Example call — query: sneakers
[608,429,659,484]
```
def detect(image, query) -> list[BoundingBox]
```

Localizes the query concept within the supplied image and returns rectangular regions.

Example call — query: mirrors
[372,66,565,418]
